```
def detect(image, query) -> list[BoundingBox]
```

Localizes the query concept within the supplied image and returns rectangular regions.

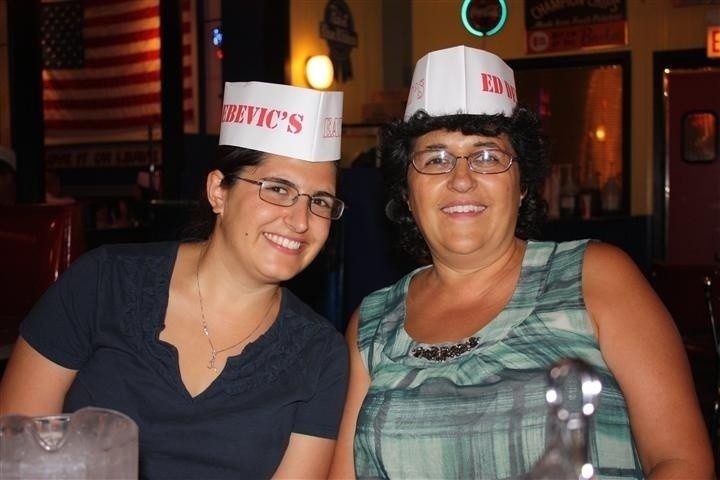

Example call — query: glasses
[235,177,345,220]
[407,149,521,175]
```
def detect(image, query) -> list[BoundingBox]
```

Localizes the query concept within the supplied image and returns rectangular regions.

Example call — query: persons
[1,82,350,480]
[326,45,716,480]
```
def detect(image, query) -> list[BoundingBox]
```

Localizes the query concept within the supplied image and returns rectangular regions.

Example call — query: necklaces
[193,256,274,370]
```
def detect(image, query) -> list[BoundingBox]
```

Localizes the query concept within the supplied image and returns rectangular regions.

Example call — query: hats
[219,80,343,162]
[404,45,517,120]
